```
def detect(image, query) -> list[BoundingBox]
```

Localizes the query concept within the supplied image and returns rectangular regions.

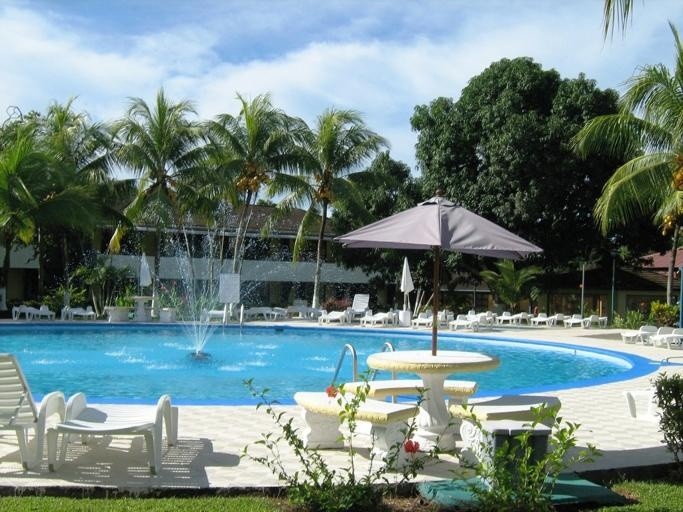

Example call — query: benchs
[464,395,562,426]
[292,390,421,461]
[341,380,478,436]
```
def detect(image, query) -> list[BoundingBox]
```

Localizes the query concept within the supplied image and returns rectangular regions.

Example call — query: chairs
[0,353,66,471]
[203,294,400,325]
[620,325,682,349]
[12,304,98,321]
[45,392,179,475]
[410,307,608,334]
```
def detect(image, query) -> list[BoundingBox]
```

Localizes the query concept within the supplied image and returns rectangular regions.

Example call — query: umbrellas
[399,256,414,311]
[332,189,544,357]
[139,252,152,296]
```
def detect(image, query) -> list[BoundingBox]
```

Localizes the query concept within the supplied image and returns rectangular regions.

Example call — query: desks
[124,295,153,321]
[366,350,500,452]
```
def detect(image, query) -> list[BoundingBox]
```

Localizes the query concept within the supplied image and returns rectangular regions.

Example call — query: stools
[459,418,551,471]
[103,305,176,322]
